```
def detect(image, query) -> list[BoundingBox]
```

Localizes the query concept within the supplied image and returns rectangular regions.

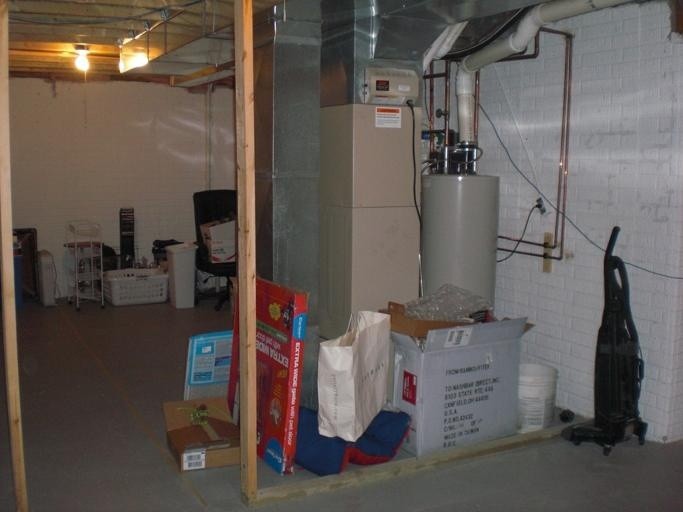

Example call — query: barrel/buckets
[517,363,560,433]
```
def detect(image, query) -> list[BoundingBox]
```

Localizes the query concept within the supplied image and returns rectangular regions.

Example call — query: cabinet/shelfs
[66,221,106,309]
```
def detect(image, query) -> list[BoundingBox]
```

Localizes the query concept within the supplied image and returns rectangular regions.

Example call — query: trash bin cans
[165,244,198,309]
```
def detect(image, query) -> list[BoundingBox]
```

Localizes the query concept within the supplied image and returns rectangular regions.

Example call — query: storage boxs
[378,309,536,459]
[199,216,236,263]
[160,397,241,472]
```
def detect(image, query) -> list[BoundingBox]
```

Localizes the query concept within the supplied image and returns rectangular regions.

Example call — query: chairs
[193,189,236,311]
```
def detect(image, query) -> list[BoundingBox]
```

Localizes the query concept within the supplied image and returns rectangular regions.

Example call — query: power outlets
[535,194,554,218]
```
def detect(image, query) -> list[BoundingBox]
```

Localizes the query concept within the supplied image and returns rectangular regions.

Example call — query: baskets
[104,267,168,305]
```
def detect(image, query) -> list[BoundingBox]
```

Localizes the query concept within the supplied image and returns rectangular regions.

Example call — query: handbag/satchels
[316,310,390,443]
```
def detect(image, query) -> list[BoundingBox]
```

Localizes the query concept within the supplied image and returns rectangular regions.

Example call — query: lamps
[75,45,89,70]
